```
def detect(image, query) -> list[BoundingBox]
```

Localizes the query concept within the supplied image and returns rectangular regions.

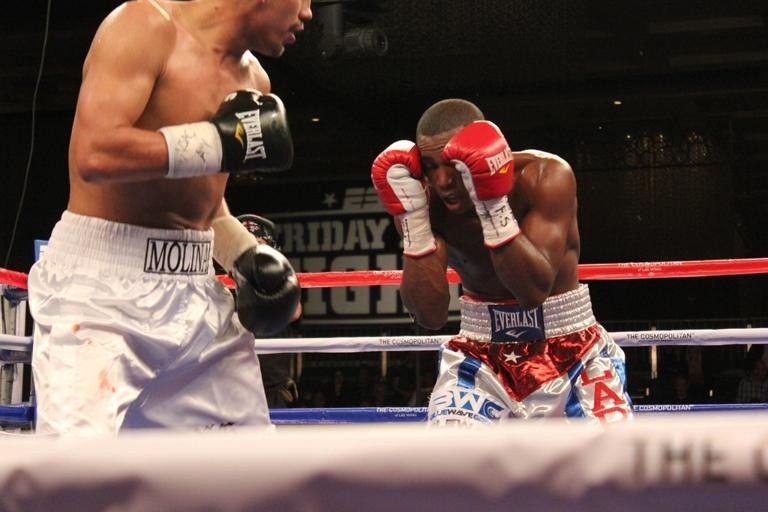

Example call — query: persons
[627,344,766,405]
[264,366,434,408]
[225,212,307,410]
[23,0,317,434]
[368,98,639,431]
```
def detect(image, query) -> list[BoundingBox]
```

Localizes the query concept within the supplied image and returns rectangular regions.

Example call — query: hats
[237,214,276,245]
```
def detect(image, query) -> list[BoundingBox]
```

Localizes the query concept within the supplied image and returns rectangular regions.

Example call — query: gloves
[157,89,294,178]
[442,120,522,249]
[210,216,299,336]
[371,140,438,257]
